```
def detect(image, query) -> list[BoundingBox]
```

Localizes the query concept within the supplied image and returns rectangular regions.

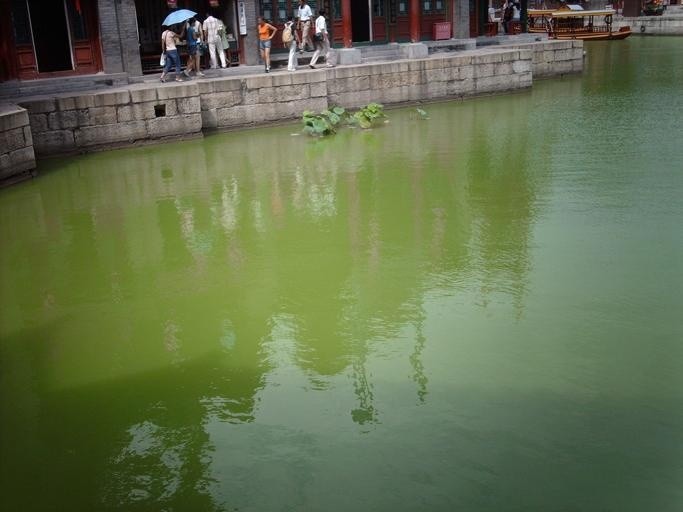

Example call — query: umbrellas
[161,8,198,27]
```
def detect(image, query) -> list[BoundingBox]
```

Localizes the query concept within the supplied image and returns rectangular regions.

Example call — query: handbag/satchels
[313,33,323,41]
[185,46,197,55]
[222,36,230,50]
[160,51,166,66]
[199,43,208,50]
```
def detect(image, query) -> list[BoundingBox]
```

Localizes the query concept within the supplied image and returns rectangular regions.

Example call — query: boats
[510,0,634,41]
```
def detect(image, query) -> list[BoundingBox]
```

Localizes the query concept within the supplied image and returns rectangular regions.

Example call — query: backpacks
[504,6,514,21]
[282,22,294,43]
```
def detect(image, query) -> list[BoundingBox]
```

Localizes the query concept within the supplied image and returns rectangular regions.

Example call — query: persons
[182,16,204,72]
[487,0,536,35]
[180,18,205,78]
[201,9,227,69]
[216,18,230,67]
[255,15,277,73]
[158,22,185,82]
[294,0,316,53]
[281,15,301,73]
[308,8,336,69]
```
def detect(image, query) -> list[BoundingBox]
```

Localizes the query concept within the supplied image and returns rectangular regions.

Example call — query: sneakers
[288,64,336,71]
[299,49,314,54]
[160,70,205,83]
[210,63,227,69]
[266,66,271,72]
